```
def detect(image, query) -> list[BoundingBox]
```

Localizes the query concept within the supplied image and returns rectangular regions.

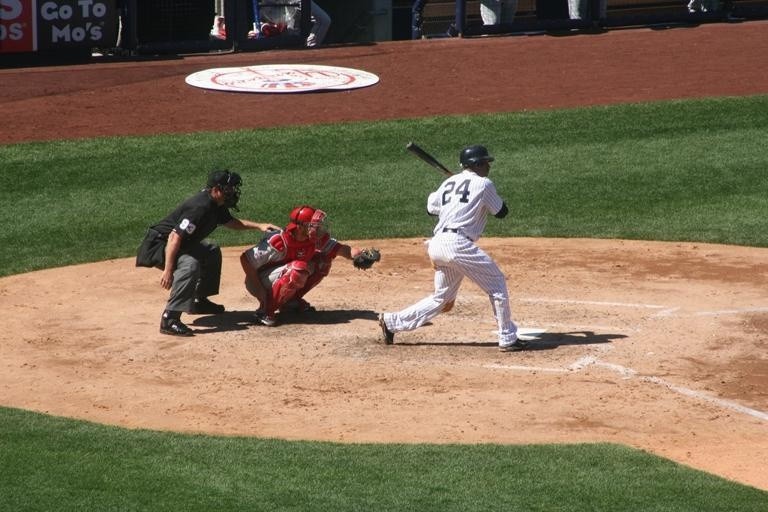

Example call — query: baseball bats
[406,142,454,176]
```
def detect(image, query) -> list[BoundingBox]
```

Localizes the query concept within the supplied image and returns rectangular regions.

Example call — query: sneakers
[158,311,194,338]
[497,338,531,353]
[278,300,315,314]
[377,312,395,346]
[185,298,225,315]
[252,309,275,327]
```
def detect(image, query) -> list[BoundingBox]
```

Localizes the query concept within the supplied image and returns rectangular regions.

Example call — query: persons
[240,205,381,326]
[378,144,533,354]
[284,1,332,46]
[134,169,282,336]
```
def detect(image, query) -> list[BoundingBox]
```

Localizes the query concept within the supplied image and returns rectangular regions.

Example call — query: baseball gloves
[355,249,380,269]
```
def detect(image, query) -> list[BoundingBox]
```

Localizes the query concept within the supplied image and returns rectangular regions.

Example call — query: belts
[441,227,474,243]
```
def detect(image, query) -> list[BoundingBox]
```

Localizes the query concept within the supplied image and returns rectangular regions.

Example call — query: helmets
[204,169,243,214]
[286,205,329,240]
[458,145,495,167]
